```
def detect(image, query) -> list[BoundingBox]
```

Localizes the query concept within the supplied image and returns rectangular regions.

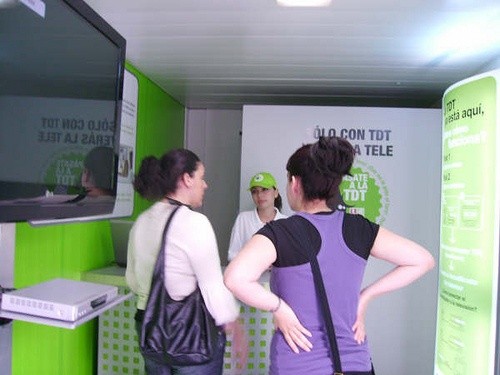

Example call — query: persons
[228,172,289,272]
[225,136,436,375]
[125,150,248,375]
[80,146,118,198]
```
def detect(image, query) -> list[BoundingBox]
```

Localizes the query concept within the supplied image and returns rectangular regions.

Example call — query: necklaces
[312,207,324,210]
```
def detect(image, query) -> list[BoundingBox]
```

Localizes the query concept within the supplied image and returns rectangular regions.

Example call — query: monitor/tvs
[0,0,126,223]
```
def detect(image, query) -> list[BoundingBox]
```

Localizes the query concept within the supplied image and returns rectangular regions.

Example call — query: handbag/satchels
[139,205,215,365]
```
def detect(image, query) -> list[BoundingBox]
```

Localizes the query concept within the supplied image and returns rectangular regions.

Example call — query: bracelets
[269,296,281,314]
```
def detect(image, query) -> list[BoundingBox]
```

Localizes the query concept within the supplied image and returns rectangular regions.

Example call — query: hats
[247,171,277,191]
